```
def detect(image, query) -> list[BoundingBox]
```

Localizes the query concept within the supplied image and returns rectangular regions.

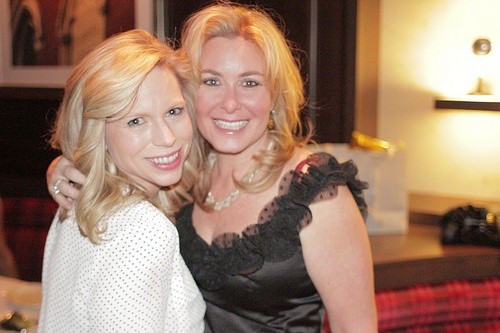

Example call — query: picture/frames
[0,0,159,105]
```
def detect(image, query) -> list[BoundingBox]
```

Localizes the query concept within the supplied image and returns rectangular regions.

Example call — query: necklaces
[202,139,276,210]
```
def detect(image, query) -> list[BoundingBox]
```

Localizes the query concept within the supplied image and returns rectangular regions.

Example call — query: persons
[39,29,207,333]
[46,0,378,332]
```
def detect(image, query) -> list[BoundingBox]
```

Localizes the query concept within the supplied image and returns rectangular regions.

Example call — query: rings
[54,180,63,194]
